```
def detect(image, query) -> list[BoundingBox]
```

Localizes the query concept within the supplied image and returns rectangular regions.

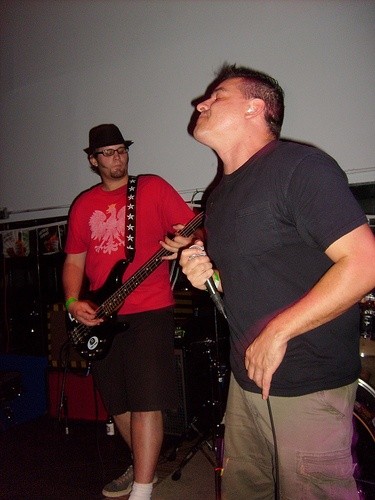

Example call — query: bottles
[106,416,116,437]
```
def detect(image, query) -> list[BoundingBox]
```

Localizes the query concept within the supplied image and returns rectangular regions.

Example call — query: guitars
[64,211,206,362]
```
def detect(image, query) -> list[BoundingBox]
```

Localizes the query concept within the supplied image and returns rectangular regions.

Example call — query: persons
[45,228,60,253]
[179,67,375,500]
[63,124,205,500]
[15,233,27,258]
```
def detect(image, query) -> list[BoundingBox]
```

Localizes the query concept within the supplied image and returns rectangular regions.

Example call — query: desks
[48,369,109,422]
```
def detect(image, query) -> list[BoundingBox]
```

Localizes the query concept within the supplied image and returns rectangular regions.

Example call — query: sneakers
[102,465,158,497]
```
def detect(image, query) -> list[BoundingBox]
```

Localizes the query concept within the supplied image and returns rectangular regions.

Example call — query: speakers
[164,347,194,438]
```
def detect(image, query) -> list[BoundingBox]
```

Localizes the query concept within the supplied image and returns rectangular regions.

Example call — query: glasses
[95,147,129,157]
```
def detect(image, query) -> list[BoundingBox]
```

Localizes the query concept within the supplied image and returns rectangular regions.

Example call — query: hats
[83,124,134,155]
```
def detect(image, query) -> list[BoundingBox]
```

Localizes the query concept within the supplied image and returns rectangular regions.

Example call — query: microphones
[188,244,228,321]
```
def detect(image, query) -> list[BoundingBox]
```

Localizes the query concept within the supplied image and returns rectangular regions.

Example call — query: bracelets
[205,272,221,292]
[65,298,80,311]
